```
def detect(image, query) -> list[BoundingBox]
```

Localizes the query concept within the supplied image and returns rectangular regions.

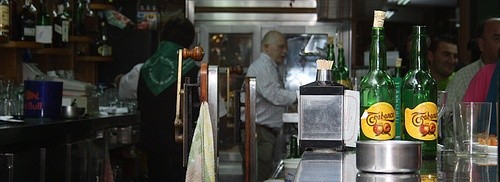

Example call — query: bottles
[0,79,22,118]
[336,48,348,86]
[359,26,396,140]
[0,0,108,56]
[400,25,437,160]
[328,44,337,83]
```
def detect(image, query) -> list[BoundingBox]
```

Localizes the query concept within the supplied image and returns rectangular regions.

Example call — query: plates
[475,144,498,155]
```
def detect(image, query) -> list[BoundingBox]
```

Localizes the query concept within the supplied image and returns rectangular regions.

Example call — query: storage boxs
[25,79,64,120]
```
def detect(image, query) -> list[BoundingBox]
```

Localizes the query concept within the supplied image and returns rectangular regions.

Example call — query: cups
[453,102,491,158]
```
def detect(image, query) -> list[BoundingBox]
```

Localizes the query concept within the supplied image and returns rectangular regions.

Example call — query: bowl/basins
[355,141,424,173]
[62,106,85,118]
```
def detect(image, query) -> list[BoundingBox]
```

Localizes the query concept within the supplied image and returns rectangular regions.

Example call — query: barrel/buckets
[24,80,63,117]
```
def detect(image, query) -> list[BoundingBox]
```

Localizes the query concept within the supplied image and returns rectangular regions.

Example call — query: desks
[0,113,134,182]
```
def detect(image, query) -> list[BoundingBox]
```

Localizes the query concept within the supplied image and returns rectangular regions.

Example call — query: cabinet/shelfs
[0,3,118,85]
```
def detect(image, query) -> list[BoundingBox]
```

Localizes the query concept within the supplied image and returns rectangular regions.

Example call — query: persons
[441,18,500,182]
[219,92,227,144]
[240,31,298,182]
[427,39,459,92]
[118,15,202,182]
